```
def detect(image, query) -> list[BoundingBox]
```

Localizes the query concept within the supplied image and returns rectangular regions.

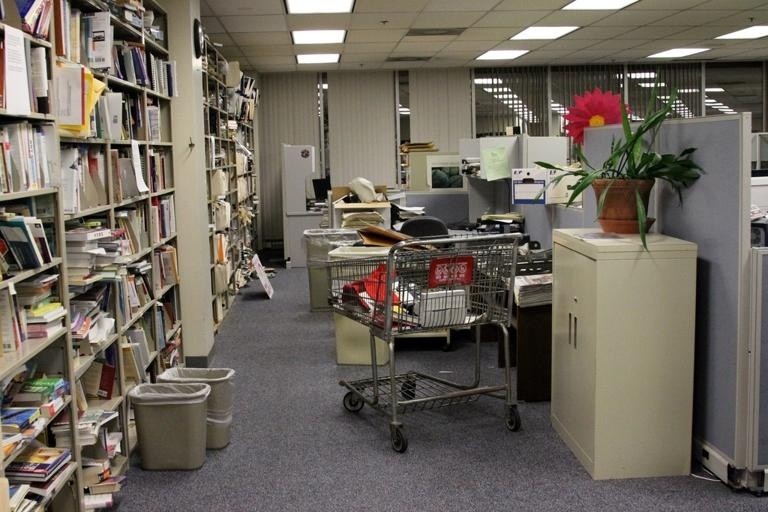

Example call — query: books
[512,273,552,308]
[202,43,258,326]
[0,0,183,512]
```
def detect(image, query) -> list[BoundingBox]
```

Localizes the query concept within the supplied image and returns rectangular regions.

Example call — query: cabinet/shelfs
[203,37,262,340]
[279,142,328,272]
[1,1,185,512]
[548,222,699,482]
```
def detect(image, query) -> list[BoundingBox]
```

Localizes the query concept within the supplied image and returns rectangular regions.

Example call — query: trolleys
[324,231,523,453]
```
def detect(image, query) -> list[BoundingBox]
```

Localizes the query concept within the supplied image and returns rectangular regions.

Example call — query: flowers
[557,90,632,149]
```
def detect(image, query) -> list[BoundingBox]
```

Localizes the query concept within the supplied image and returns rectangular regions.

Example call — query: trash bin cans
[328,246,390,365]
[304,228,357,312]
[127,366,235,470]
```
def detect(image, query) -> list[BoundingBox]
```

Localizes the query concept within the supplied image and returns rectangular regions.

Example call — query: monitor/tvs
[312,179,331,200]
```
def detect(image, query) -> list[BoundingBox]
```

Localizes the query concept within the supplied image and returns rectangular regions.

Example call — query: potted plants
[529,83,707,255]
